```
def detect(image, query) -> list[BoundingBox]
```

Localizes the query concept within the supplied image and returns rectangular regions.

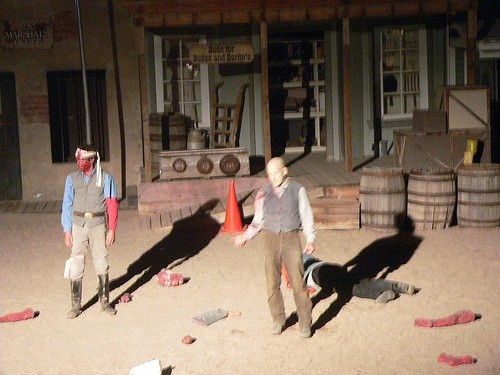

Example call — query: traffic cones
[218,180,249,234]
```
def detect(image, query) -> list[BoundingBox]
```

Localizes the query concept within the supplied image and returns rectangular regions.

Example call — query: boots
[97,273,116,315]
[67,280,82,318]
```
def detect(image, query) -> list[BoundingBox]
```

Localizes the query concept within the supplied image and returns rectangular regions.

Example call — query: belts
[73,211,105,218]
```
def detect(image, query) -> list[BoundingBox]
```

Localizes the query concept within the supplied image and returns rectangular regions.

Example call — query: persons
[230,157,316,338]
[302,252,415,304]
[61,144,120,319]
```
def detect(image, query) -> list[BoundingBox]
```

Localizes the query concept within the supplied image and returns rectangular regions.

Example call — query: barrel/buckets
[359,166,406,232]
[406,168,457,231]
[162,114,186,150]
[456,163,500,228]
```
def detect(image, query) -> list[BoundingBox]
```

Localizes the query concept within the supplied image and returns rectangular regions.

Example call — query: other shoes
[299,324,311,337]
[270,322,285,334]
[376,290,395,304]
[404,283,415,294]
